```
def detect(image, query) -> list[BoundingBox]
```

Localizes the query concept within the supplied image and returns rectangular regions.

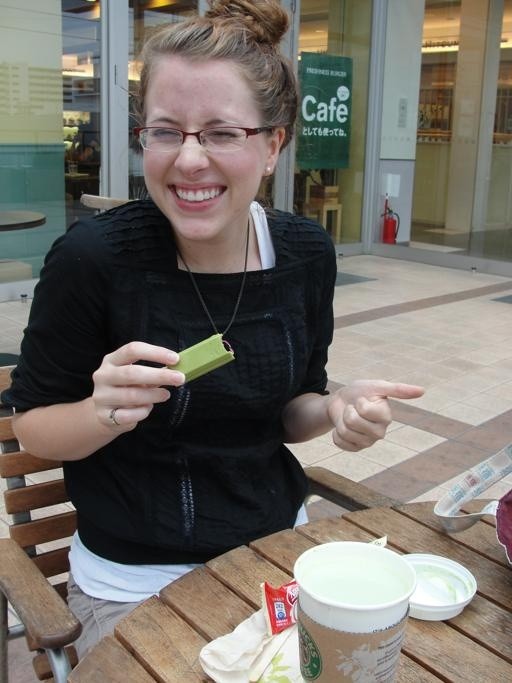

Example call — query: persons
[65,124,101,200]
[1,0,429,663]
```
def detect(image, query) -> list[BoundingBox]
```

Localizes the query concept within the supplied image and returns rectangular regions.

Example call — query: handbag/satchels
[433,442,512,567]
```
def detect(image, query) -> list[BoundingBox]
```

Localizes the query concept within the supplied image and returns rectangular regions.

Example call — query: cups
[292,540,417,683]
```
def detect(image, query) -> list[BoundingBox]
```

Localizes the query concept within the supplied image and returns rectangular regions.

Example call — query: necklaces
[173,217,252,354]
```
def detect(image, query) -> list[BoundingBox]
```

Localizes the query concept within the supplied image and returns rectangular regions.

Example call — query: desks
[65,498,511,683]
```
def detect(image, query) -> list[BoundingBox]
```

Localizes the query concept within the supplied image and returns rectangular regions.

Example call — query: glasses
[132,124,275,151]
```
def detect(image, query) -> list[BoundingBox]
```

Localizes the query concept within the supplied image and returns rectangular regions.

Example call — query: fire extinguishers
[380,207,400,243]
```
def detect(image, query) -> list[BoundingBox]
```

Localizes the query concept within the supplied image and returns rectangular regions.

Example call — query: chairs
[0,365,410,678]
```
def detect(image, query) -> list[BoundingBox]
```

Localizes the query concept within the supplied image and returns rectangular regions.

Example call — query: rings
[107,407,121,427]
